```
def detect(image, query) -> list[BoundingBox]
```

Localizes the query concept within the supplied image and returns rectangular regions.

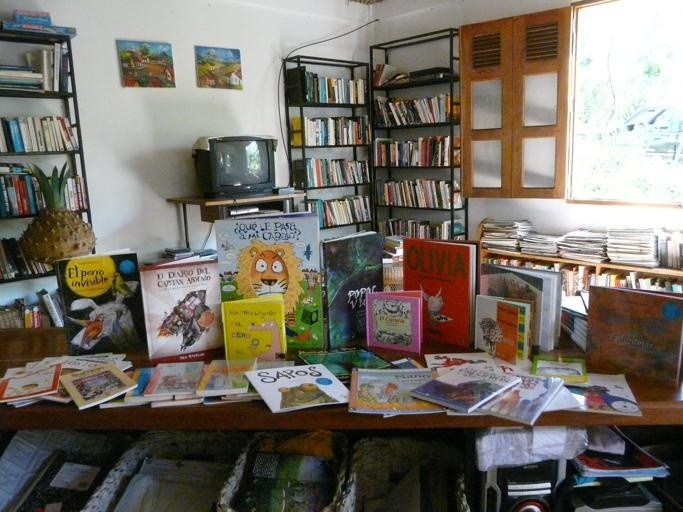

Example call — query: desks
[165,190,306,252]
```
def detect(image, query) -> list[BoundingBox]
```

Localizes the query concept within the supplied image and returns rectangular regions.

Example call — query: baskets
[336,438,470,512]
[216,429,346,512]
[79,428,248,512]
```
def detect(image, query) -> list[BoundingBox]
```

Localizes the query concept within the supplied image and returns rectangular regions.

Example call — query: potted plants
[20,159,98,264]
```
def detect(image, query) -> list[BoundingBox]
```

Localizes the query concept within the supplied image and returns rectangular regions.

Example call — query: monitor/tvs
[195,136,275,198]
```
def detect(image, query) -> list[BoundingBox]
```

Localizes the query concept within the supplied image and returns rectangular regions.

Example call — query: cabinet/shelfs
[474,218,683,302]
[283,55,371,231]
[370,25,469,243]
[0,29,98,326]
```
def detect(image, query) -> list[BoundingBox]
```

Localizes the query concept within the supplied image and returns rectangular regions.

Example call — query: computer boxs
[481,433,568,512]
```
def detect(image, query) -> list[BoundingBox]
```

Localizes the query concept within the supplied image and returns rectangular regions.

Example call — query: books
[0,9,89,215]
[285,58,467,243]
